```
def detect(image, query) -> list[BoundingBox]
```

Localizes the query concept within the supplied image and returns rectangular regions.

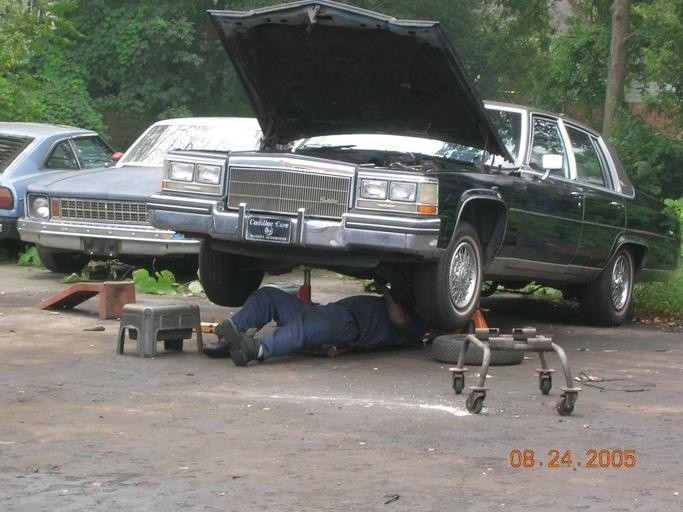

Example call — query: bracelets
[379,287,386,292]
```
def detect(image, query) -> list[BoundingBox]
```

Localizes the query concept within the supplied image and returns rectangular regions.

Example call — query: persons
[200,278,428,368]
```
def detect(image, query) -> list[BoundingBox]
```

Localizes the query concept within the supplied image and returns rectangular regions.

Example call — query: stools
[113,298,205,358]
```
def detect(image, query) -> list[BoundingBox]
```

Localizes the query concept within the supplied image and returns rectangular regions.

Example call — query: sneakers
[201,338,232,359]
[217,315,259,368]
